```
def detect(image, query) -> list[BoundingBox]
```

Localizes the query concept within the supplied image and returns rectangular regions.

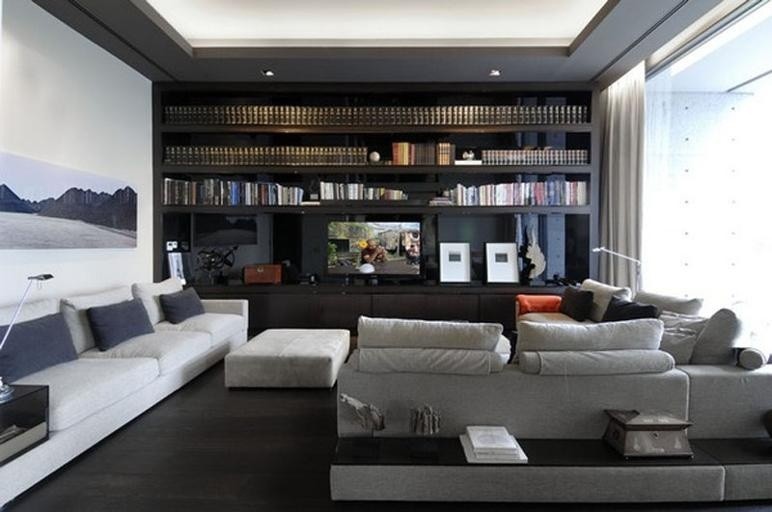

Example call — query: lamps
[0,273,53,400]
[592,244,642,288]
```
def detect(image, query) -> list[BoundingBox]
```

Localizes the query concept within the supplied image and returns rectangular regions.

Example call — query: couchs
[337,278,772,437]
[0,280,249,507]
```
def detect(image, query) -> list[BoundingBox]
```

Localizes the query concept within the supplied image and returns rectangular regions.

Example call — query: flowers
[359,241,368,249]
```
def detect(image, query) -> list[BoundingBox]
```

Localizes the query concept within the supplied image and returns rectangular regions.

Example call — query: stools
[223,329,349,390]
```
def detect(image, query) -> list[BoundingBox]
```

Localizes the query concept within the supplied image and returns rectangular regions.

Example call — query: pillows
[0,312,78,384]
[61,286,134,354]
[88,299,154,351]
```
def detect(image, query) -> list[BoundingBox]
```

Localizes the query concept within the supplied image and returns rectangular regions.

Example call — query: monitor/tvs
[325,217,423,278]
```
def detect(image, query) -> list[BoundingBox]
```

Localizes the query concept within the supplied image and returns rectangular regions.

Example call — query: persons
[361,239,386,264]
[406,245,420,264]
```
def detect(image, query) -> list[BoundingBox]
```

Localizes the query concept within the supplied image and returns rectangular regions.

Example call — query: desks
[330,440,771,503]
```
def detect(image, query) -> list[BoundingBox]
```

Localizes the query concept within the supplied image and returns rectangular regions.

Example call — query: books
[459,424,528,464]
[162,105,589,205]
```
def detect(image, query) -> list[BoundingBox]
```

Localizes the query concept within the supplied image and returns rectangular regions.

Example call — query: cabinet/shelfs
[150,81,599,338]
